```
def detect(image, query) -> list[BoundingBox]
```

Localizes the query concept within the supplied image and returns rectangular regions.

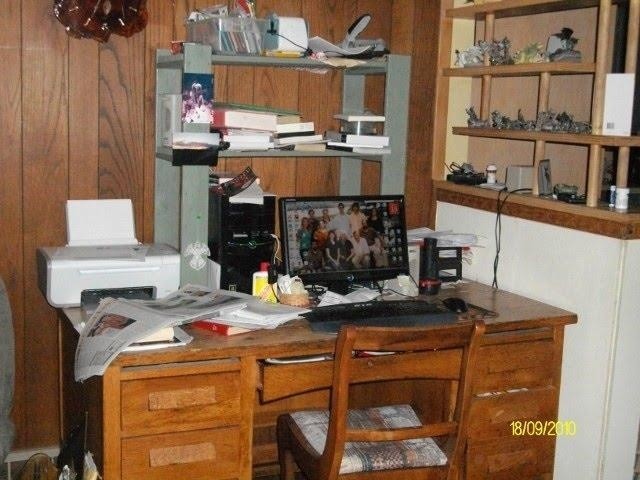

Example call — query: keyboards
[298,299,459,332]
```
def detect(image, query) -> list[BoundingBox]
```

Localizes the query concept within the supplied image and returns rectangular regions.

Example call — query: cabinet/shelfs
[153,41,412,295]
[441,0,640,214]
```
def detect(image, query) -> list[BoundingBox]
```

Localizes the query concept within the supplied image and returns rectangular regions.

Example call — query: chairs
[273,319,486,478]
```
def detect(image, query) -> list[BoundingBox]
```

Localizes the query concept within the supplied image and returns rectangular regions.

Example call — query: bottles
[609,184,630,210]
[486,163,496,186]
[422,236,441,294]
[252,263,273,297]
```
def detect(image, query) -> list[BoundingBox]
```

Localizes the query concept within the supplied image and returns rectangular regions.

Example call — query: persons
[296,203,388,270]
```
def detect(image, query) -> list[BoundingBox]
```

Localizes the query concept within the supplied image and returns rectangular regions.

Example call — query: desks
[58,270,577,479]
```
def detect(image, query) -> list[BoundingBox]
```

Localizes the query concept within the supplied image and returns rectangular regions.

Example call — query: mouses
[442,297,468,313]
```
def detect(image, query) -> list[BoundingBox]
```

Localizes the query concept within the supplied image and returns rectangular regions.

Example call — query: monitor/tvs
[278,194,410,296]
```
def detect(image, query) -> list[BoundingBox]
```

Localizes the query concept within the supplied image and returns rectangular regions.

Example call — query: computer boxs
[209,189,276,295]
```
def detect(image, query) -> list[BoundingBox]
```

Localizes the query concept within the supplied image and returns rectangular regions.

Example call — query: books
[135,326,175,342]
[192,321,256,336]
[211,103,392,155]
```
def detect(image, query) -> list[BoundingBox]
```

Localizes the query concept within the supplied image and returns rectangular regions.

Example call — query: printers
[36,199,180,315]
